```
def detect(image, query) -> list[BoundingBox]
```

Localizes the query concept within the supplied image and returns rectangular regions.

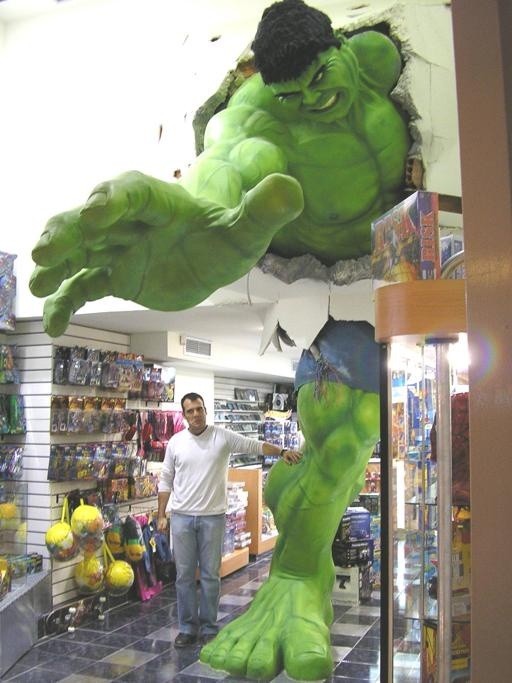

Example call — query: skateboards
[46,589,128,634]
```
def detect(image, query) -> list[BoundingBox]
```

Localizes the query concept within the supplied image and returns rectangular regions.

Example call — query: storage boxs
[332,563,372,608]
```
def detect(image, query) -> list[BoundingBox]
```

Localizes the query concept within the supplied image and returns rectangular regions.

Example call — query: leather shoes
[175,632,197,647]
[200,633,217,647]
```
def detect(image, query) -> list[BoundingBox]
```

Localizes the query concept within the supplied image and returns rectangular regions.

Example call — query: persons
[157,393,303,648]
[27,0,408,677]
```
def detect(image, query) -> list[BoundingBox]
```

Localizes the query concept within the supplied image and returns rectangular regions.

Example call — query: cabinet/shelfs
[374,0,512,683]
[358,456,418,594]
[182,482,249,579]
[228,467,278,556]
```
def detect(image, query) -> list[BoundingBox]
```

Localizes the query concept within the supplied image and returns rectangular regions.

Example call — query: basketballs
[45,505,135,597]
